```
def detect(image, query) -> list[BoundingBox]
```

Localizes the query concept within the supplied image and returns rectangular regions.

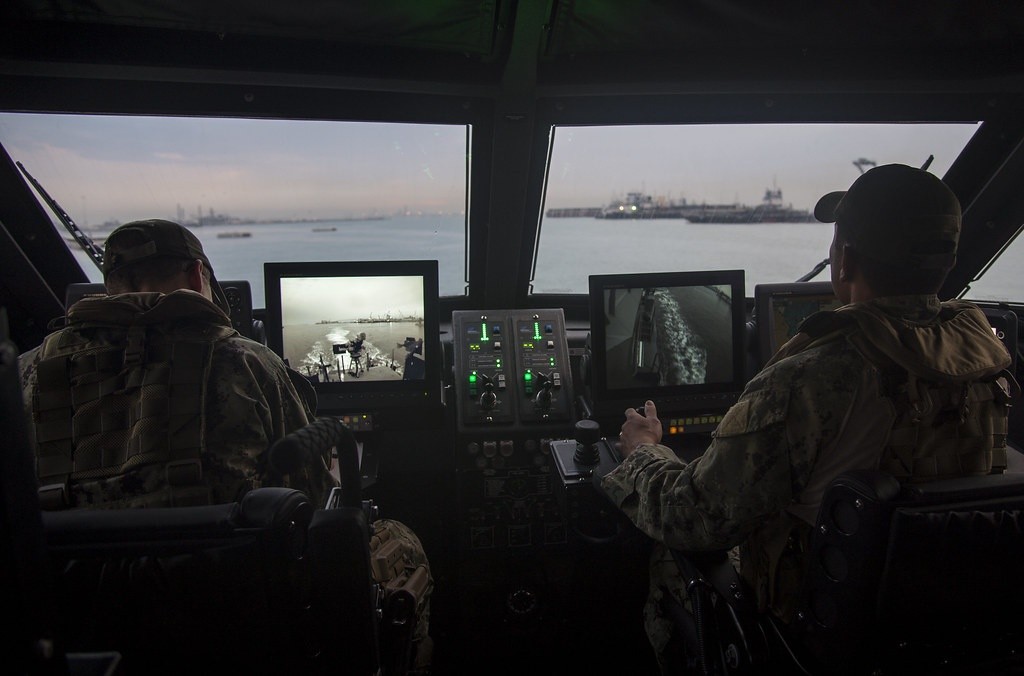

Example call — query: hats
[103,219,232,316]
[813,164,962,270]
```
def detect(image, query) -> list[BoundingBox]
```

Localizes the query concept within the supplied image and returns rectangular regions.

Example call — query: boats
[599,187,816,224]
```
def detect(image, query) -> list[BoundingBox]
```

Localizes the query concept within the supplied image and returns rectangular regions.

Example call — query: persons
[591,160,1012,676]
[11,216,437,676]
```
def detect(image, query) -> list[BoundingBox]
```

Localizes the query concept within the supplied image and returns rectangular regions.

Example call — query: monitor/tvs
[264,260,439,406]
[589,268,746,414]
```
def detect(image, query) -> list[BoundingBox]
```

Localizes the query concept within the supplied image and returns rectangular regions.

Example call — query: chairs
[666,442,1024,676]
[1,341,418,676]
